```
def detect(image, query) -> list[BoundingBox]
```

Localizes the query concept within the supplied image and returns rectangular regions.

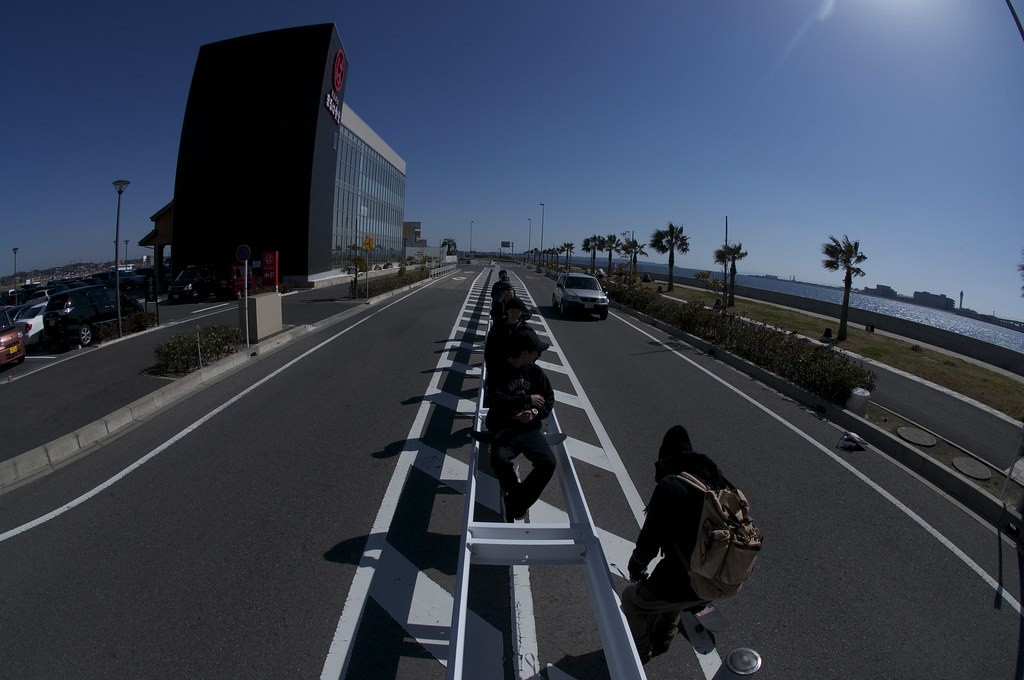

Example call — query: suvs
[43,284,144,345]
[553,272,609,320]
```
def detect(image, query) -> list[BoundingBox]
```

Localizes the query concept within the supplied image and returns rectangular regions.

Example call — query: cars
[0,268,146,345]
[0,307,26,369]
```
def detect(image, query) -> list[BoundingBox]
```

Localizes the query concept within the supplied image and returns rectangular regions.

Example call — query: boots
[652,620,678,653]
[621,606,650,665]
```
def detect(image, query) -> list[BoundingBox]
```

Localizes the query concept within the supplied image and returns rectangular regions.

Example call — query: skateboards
[689,601,731,633]
[464,428,567,446]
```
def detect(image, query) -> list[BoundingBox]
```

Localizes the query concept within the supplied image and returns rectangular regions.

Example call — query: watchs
[531,408,538,417]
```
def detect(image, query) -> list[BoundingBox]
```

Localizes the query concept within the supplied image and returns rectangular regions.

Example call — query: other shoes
[502,496,529,523]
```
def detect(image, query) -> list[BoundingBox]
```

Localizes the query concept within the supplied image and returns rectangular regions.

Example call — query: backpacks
[676,471,762,585]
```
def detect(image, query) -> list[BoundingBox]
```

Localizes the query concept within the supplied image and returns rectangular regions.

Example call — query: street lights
[113,179,131,338]
[124,239,130,271]
[540,203,544,264]
[470,220,474,259]
[511,241,514,262]
[528,218,531,261]
[498,248,502,258]
[12,247,19,306]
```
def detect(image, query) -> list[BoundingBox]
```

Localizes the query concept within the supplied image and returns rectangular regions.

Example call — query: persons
[596,267,603,279]
[628,424,736,665]
[483,269,533,385]
[491,330,556,523]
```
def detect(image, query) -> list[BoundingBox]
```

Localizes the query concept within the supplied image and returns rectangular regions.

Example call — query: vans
[168,264,221,303]
[222,264,258,299]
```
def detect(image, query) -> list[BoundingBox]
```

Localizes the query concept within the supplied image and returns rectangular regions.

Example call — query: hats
[658,426,693,460]
[511,330,549,353]
[507,297,523,311]
